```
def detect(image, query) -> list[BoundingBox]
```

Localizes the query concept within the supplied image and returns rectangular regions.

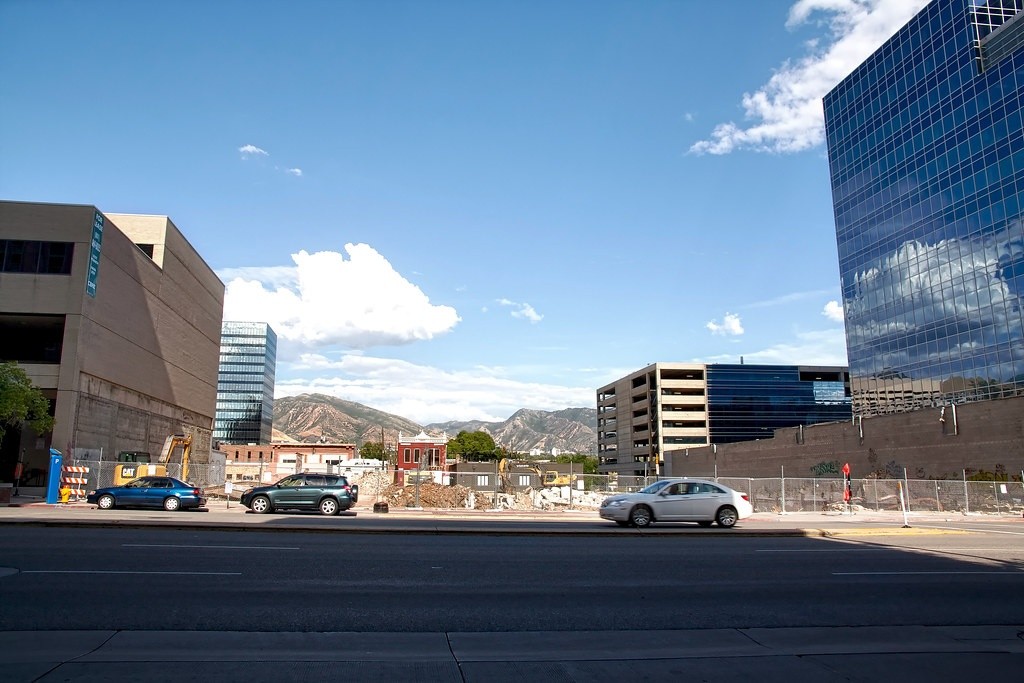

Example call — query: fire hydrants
[58,484,72,504]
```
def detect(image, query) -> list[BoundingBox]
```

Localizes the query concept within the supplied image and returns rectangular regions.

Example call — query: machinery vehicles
[498,458,578,495]
[114,432,195,489]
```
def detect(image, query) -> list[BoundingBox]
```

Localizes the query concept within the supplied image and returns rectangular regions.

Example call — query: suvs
[240,472,359,516]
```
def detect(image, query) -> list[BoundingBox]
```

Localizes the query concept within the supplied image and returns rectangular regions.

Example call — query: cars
[599,478,753,529]
[87,475,206,512]
